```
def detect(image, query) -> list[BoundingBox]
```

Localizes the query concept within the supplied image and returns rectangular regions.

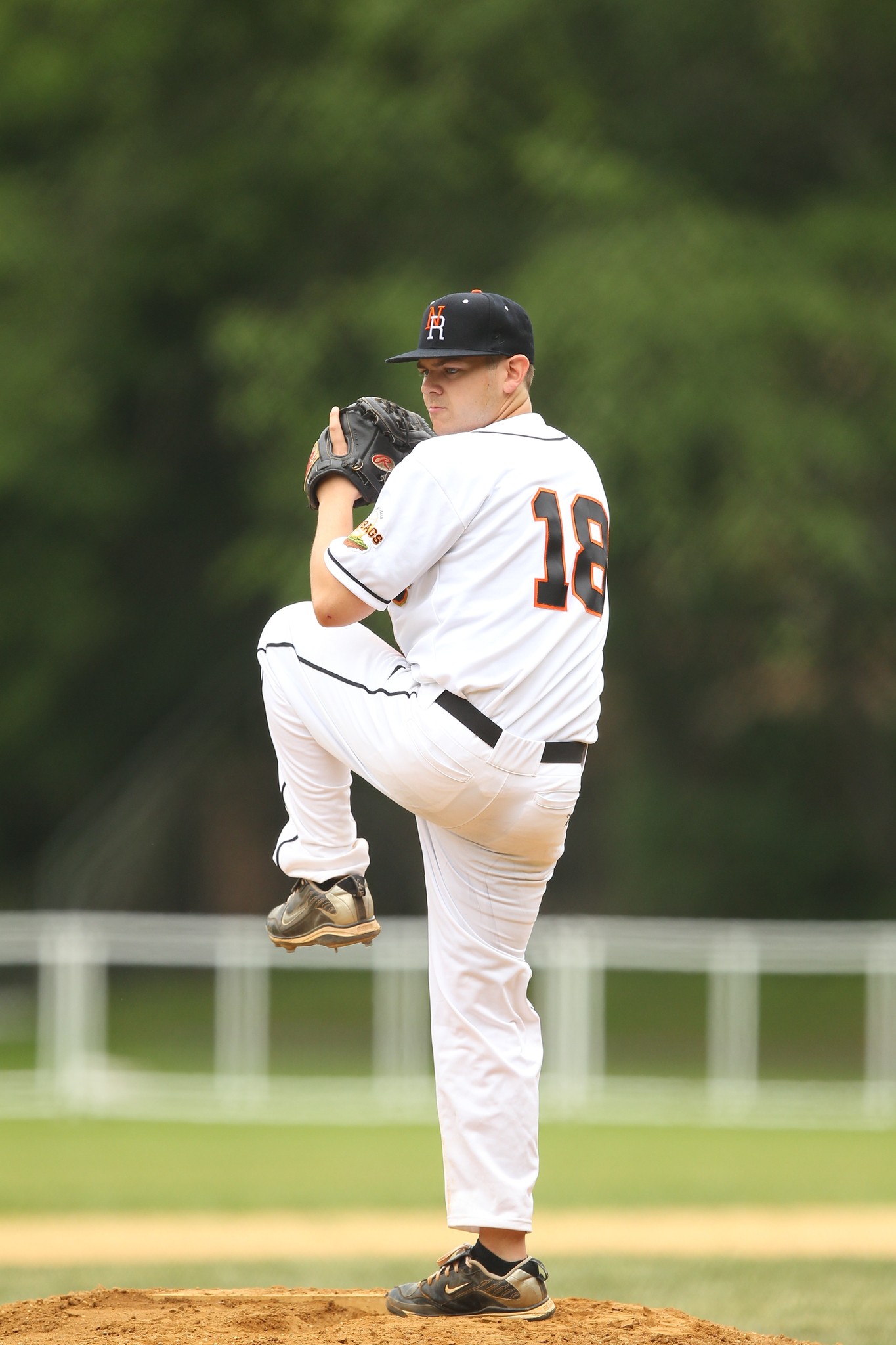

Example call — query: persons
[252,291,614,1322]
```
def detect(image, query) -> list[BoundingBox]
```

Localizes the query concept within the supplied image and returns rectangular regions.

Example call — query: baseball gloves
[301,394,440,513]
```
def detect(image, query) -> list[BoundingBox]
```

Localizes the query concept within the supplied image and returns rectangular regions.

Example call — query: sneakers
[385,1243,556,1322]
[265,874,381,953]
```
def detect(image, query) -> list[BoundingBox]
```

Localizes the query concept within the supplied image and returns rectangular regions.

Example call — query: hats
[385,289,535,365]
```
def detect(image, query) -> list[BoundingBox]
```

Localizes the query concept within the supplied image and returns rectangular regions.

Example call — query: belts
[434,689,587,764]
